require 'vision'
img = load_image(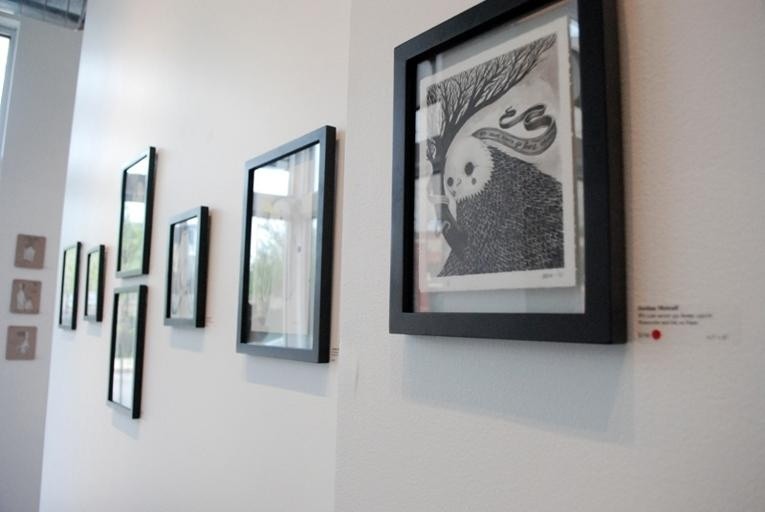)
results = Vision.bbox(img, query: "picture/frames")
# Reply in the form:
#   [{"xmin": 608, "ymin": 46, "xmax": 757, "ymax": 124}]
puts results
[
  {"xmin": 58, "ymin": 242, "xmax": 81, "ymax": 331},
  {"xmin": 164, "ymin": 206, "xmax": 208, "ymax": 329},
  {"xmin": 109, "ymin": 285, "xmax": 148, "ymax": 420},
  {"xmin": 115, "ymin": 147, "xmax": 156, "ymax": 279},
  {"xmin": 390, "ymin": 2, "xmax": 625, "ymax": 343},
  {"xmin": 83, "ymin": 245, "xmax": 105, "ymax": 323},
  {"xmin": 236, "ymin": 125, "xmax": 337, "ymax": 364}
]
